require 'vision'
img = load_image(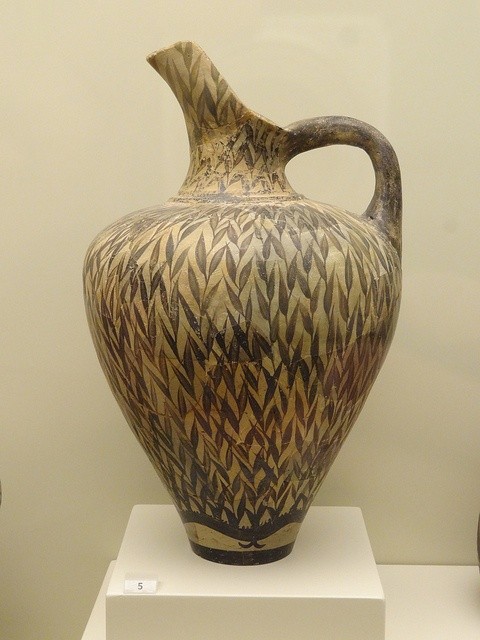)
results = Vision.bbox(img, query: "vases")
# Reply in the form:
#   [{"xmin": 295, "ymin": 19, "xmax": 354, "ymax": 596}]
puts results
[{"xmin": 80, "ymin": 42, "xmax": 403, "ymax": 567}]
